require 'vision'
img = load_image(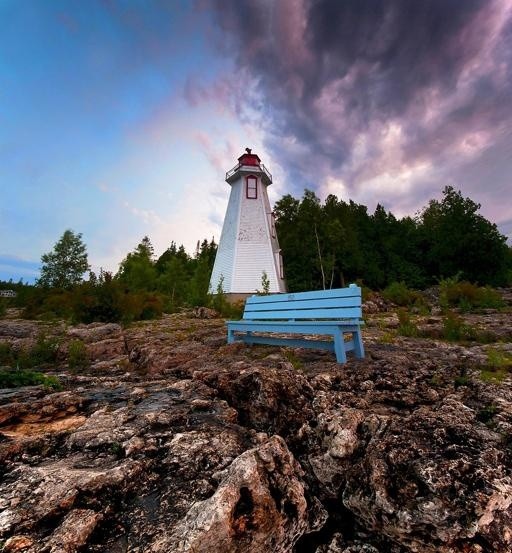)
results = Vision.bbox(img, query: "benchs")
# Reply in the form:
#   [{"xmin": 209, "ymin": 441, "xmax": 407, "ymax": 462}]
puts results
[{"xmin": 225, "ymin": 287, "xmax": 366, "ymax": 363}]
